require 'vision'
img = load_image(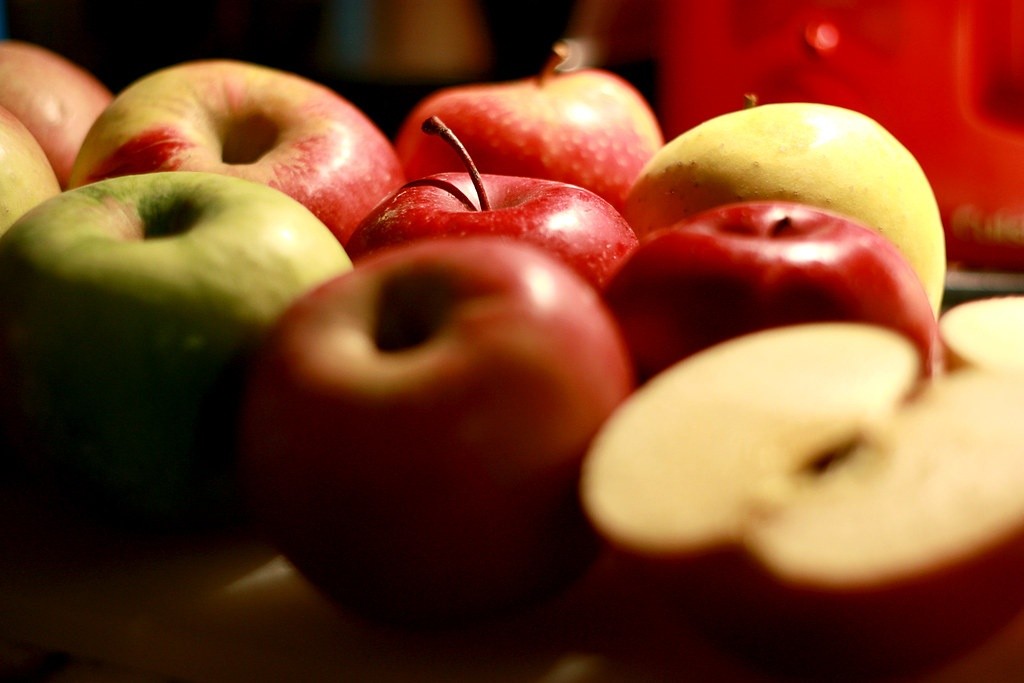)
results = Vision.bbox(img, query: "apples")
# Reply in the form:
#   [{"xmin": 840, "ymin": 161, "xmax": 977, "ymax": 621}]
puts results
[{"xmin": 0, "ymin": 41, "xmax": 1024, "ymax": 673}]
